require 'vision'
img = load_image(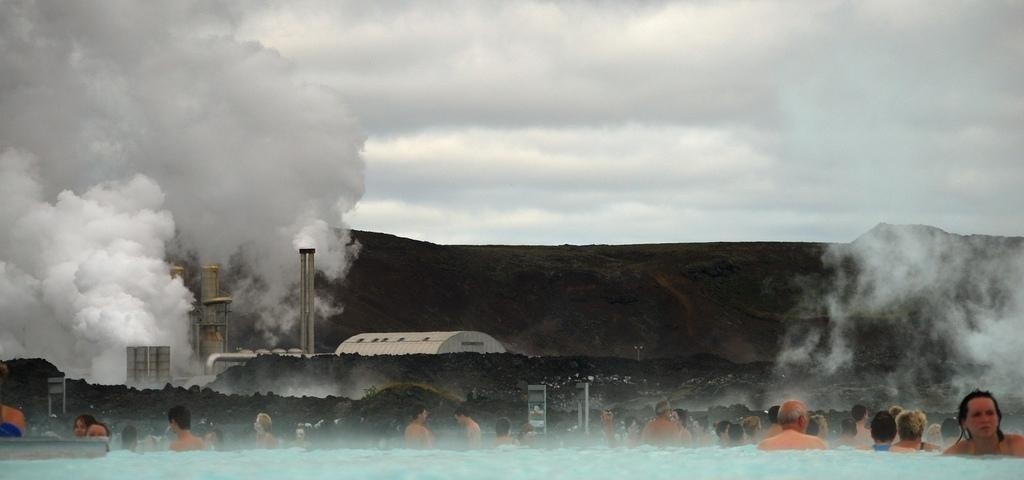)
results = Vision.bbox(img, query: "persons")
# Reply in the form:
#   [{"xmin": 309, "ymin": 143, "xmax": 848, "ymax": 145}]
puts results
[
  {"xmin": 121, "ymin": 425, "xmax": 138, "ymax": 454},
  {"xmin": 254, "ymin": 412, "xmax": 278, "ymax": 450},
  {"xmin": 84, "ymin": 422, "xmax": 110, "ymax": 451},
  {"xmin": 71, "ymin": 414, "xmax": 95, "ymax": 439},
  {"xmin": 0, "ymin": 362, "xmax": 24, "ymax": 439},
  {"xmin": 169, "ymin": 408, "xmax": 205, "ymax": 453},
  {"xmin": 516, "ymin": 422, "xmax": 537, "ymax": 447},
  {"xmin": 208, "ymin": 428, "xmax": 225, "ymax": 453},
  {"xmin": 404, "ymin": 404, "xmax": 433, "ymax": 451},
  {"xmin": 491, "ymin": 416, "xmax": 519, "ymax": 451},
  {"xmin": 598, "ymin": 386, "xmax": 1023, "ymax": 457},
  {"xmin": 454, "ymin": 407, "xmax": 483, "ymax": 451},
  {"xmin": 142, "ymin": 436, "xmax": 161, "ymax": 452}
]
